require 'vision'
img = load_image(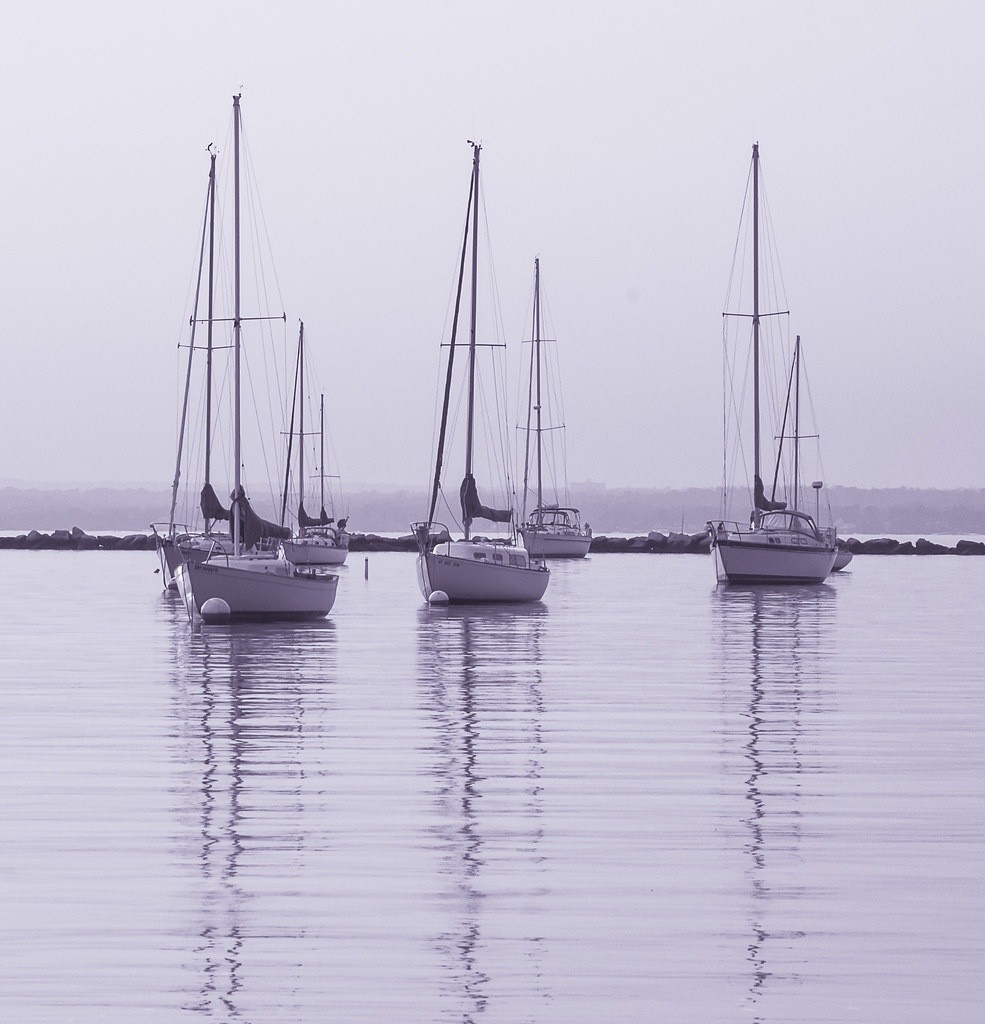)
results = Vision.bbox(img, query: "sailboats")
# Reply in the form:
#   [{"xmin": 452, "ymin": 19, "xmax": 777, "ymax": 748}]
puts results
[
  {"xmin": 771, "ymin": 334, "xmax": 853, "ymax": 572},
  {"xmin": 410, "ymin": 140, "xmax": 551, "ymax": 601},
  {"xmin": 706, "ymin": 141, "xmax": 838, "ymax": 585},
  {"xmin": 517, "ymin": 258, "xmax": 594, "ymax": 558},
  {"xmin": 149, "ymin": 154, "xmax": 287, "ymax": 589},
  {"xmin": 176, "ymin": 92, "xmax": 340, "ymax": 619}
]
